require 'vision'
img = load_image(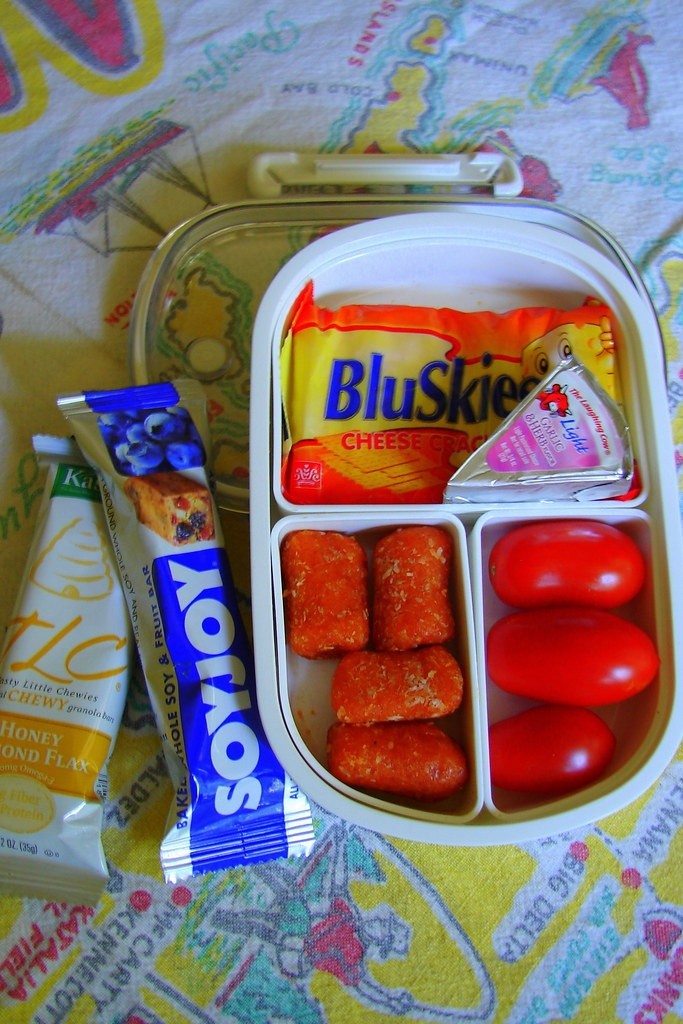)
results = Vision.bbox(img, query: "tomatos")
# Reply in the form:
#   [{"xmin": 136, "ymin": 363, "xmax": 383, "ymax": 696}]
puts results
[
  {"xmin": 489, "ymin": 521, "xmax": 643, "ymax": 608},
  {"xmin": 485, "ymin": 608, "xmax": 658, "ymax": 706},
  {"xmin": 488, "ymin": 708, "xmax": 615, "ymax": 793}
]
